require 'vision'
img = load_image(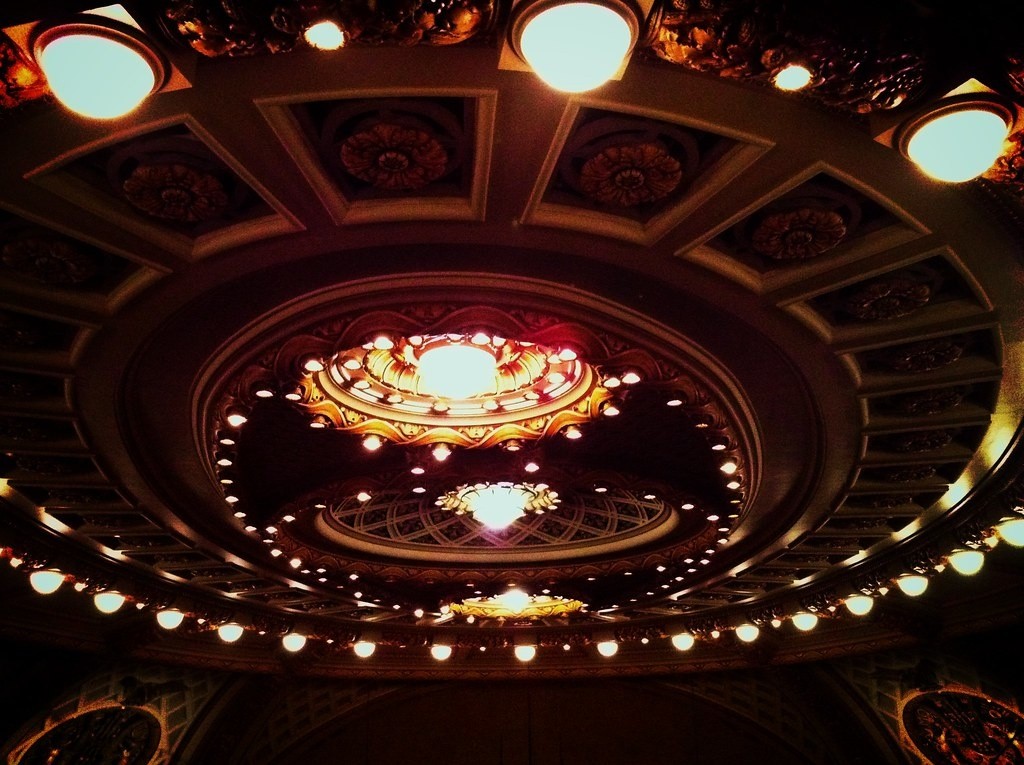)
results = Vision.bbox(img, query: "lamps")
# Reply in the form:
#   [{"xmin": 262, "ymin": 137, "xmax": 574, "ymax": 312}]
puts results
[{"xmin": 1, "ymin": 0, "xmax": 1024, "ymax": 664}]
